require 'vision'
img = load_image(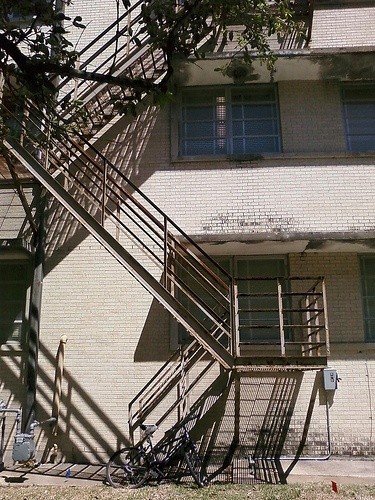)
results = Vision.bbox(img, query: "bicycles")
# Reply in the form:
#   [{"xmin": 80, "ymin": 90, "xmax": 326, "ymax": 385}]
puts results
[{"xmin": 107, "ymin": 413, "xmax": 211, "ymax": 489}]
